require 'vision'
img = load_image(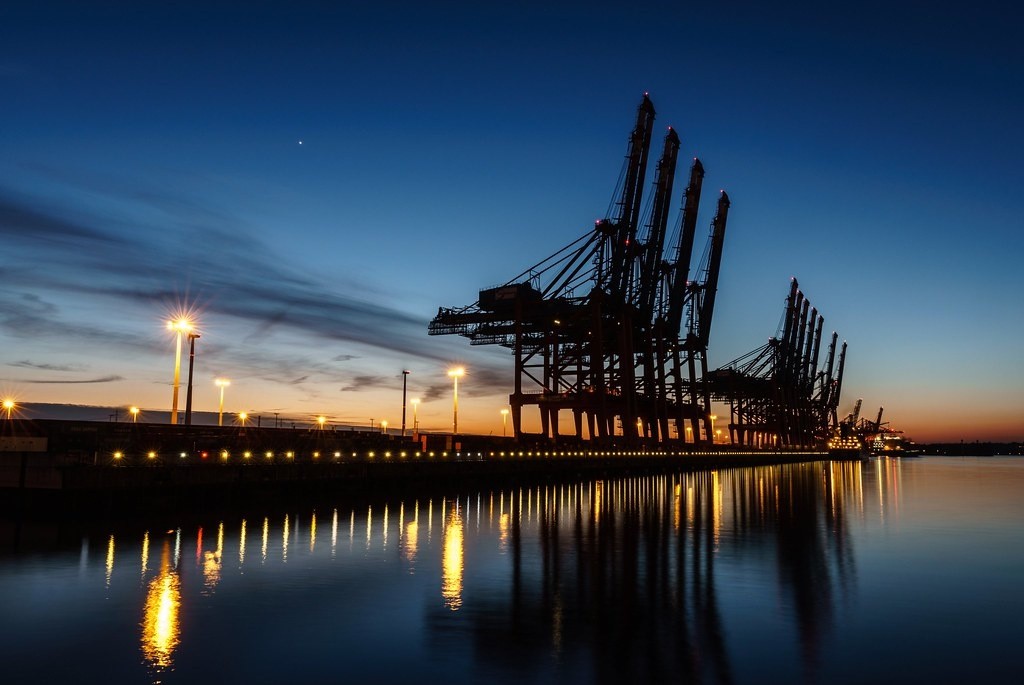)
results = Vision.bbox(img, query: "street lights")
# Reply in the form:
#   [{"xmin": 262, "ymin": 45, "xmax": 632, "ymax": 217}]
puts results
[
  {"xmin": 2, "ymin": 399, "xmax": 14, "ymax": 422},
  {"xmin": 446, "ymin": 367, "xmax": 465, "ymax": 435},
  {"xmin": 164, "ymin": 321, "xmax": 191, "ymax": 424},
  {"xmin": 126, "ymin": 405, "xmax": 140, "ymax": 424},
  {"xmin": 316, "ymin": 415, "xmax": 327, "ymax": 432},
  {"xmin": 409, "ymin": 397, "xmax": 422, "ymax": 436},
  {"xmin": 216, "ymin": 377, "xmax": 230, "ymax": 427},
  {"xmin": 240, "ymin": 412, "xmax": 248, "ymax": 427},
  {"xmin": 382, "ymin": 421, "xmax": 389, "ymax": 436},
  {"xmin": 499, "ymin": 407, "xmax": 510, "ymax": 438}
]
